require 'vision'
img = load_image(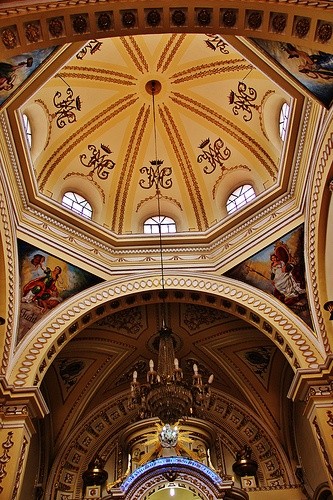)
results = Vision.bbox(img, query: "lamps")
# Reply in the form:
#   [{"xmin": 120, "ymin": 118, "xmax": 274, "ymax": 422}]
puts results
[{"xmin": 128, "ymin": 77, "xmax": 217, "ymax": 450}]
